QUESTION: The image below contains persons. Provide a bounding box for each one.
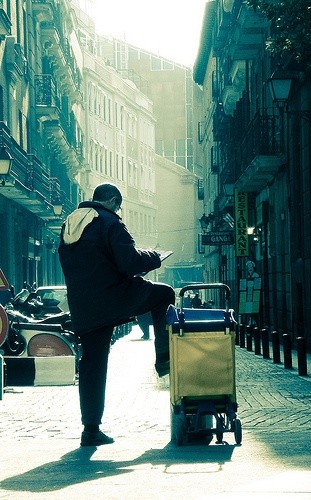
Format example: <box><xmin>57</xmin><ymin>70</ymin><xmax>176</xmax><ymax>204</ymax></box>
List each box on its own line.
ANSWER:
<box><xmin>192</xmin><ymin>293</ymin><xmax>202</xmax><ymax>309</ymax></box>
<box><xmin>58</xmin><ymin>184</ymin><xmax>176</xmax><ymax>448</ymax></box>
<box><xmin>137</xmin><ymin>312</ymin><xmax>150</xmax><ymax>339</ymax></box>
<box><xmin>183</xmin><ymin>295</ymin><xmax>192</xmax><ymax>308</ymax></box>
<box><xmin>245</xmin><ymin>260</ymin><xmax>259</xmax><ymax>278</ymax></box>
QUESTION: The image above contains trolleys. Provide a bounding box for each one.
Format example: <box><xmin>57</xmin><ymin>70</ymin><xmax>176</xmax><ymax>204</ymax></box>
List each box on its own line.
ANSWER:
<box><xmin>165</xmin><ymin>282</ymin><xmax>241</xmax><ymax>446</ymax></box>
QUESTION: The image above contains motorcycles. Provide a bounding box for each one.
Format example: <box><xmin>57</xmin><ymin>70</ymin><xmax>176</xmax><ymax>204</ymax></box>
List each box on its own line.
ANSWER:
<box><xmin>4</xmin><ymin>282</ymin><xmax>82</xmax><ymax>374</ymax></box>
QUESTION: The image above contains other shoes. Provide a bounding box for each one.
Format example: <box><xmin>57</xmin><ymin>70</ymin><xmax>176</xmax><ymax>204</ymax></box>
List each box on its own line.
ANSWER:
<box><xmin>80</xmin><ymin>430</ymin><xmax>113</xmax><ymax>446</ymax></box>
<box><xmin>156</xmin><ymin>361</ymin><xmax>169</xmax><ymax>377</ymax></box>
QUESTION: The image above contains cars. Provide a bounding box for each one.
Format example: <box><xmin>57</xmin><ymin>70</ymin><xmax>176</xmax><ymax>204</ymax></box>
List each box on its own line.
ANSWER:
<box><xmin>34</xmin><ymin>286</ymin><xmax>69</xmax><ymax>314</ymax></box>
